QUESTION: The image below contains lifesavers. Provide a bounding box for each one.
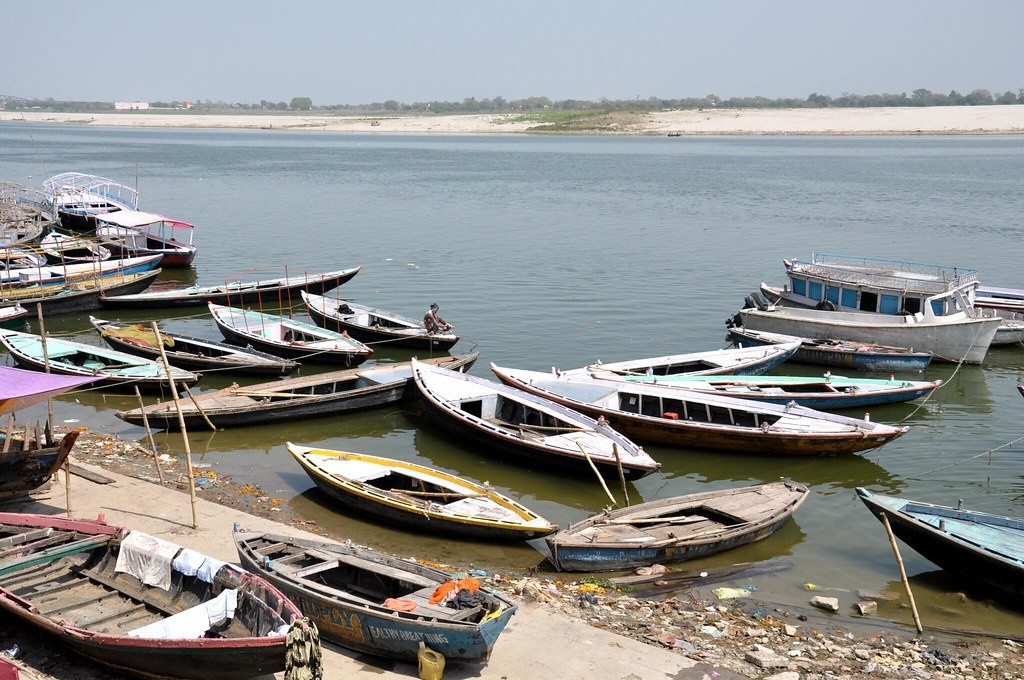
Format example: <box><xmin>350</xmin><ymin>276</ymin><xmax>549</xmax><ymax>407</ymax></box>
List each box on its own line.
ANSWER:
<box><xmin>896</xmin><ymin>311</ymin><xmax>913</xmax><ymax>316</ymax></box>
<box><xmin>815</xmin><ymin>300</ymin><xmax>836</xmax><ymax>311</ymax></box>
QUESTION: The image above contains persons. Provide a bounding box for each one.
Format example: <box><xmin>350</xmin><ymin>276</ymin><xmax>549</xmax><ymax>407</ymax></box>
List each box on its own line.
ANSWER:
<box><xmin>423</xmin><ymin>302</ymin><xmax>454</xmax><ymax>335</ymax></box>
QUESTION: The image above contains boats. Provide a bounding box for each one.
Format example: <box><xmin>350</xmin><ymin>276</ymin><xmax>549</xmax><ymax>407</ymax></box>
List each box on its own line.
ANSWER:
<box><xmin>411</xmin><ymin>356</ymin><xmax>663</xmax><ymax>482</ymax></box>
<box><xmin>0</xmin><ymin>512</ymin><xmax>311</xmax><ymax>680</ymax></box>
<box><xmin>0</xmin><ymin>171</ymin><xmax>164</xmax><ymax>330</ymax></box>
<box><xmin>98</xmin><ymin>263</ymin><xmax>362</xmax><ymax>310</ymax></box>
<box><xmin>88</xmin><ymin>315</ymin><xmax>302</xmax><ymax>377</ymax></box>
<box><xmin>301</xmin><ymin>289</ymin><xmax>461</xmax><ymax>352</ymax></box>
<box><xmin>855</xmin><ymin>486</ymin><xmax>1024</xmax><ymax>607</ymax></box>
<box><xmin>526</xmin><ymin>476</ymin><xmax>810</xmax><ymax>572</ymax></box>
<box><xmin>286</xmin><ymin>441</ymin><xmax>560</xmax><ymax>544</ymax></box>
<box><xmin>208</xmin><ymin>266</ymin><xmax>374</xmax><ymax>368</ymax></box>
<box><xmin>552</xmin><ymin>339</ymin><xmax>943</xmax><ymax>413</ymax></box>
<box><xmin>94</xmin><ymin>210</ymin><xmax>197</xmax><ymax>268</ymax></box>
<box><xmin>490</xmin><ymin>361</ymin><xmax>910</xmax><ymax>457</ymax></box>
<box><xmin>728</xmin><ymin>324</ymin><xmax>933</xmax><ymax>374</ymax></box>
<box><xmin>232</xmin><ymin>522</ymin><xmax>518</xmax><ymax>666</ymax></box>
<box><xmin>114</xmin><ymin>350</ymin><xmax>481</xmax><ymax>433</ymax></box>
<box><xmin>725</xmin><ymin>250</ymin><xmax>1024</xmax><ymax>366</ymax></box>
<box><xmin>0</xmin><ymin>366</ymin><xmax>110</xmax><ymax>500</ymax></box>
<box><xmin>0</xmin><ymin>329</ymin><xmax>203</xmax><ymax>396</ymax></box>
